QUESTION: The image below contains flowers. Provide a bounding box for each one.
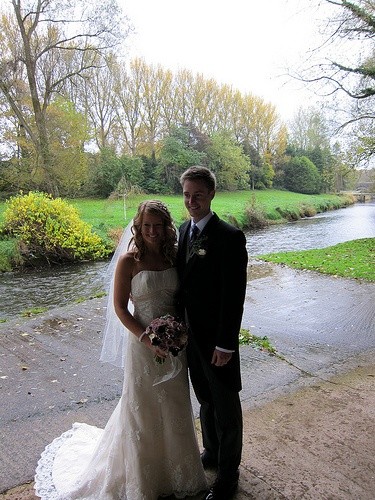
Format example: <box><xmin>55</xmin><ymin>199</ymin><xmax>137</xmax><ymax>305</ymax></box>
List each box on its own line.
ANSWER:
<box><xmin>196</xmin><ymin>248</ymin><xmax>207</xmax><ymax>257</ymax></box>
<box><xmin>139</xmin><ymin>314</ymin><xmax>188</xmax><ymax>363</ymax></box>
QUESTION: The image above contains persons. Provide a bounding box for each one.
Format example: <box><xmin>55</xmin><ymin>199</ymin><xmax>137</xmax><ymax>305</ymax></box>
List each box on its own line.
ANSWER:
<box><xmin>114</xmin><ymin>201</ymin><xmax>190</xmax><ymax>500</ymax></box>
<box><xmin>174</xmin><ymin>165</ymin><xmax>249</xmax><ymax>500</ymax></box>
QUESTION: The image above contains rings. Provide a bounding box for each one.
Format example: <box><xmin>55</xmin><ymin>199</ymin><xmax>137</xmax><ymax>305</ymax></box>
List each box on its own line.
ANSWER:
<box><xmin>222</xmin><ymin>362</ymin><xmax>227</xmax><ymax>364</ymax></box>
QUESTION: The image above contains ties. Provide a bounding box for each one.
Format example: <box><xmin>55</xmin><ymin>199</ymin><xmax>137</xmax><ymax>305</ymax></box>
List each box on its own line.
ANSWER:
<box><xmin>187</xmin><ymin>225</ymin><xmax>200</xmax><ymax>260</ymax></box>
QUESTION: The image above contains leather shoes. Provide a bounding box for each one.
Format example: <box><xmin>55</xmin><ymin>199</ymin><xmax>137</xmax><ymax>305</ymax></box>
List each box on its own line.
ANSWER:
<box><xmin>201</xmin><ymin>483</ymin><xmax>235</xmax><ymax>500</ymax></box>
<box><xmin>199</xmin><ymin>451</ymin><xmax>216</xmax><ymax>469</ymax></box>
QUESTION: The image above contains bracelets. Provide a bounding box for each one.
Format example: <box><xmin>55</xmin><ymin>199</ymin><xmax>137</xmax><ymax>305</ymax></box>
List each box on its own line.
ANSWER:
<box><xmin>139</xmin><ymin>331</ymin><xmax>147</xmax><ymax>343</ymax></box>
